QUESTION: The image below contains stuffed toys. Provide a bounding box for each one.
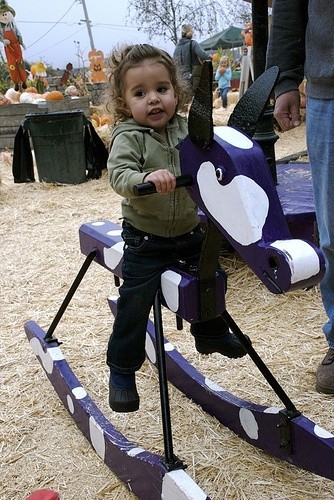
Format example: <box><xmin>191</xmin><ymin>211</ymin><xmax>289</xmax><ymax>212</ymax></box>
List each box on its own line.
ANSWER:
<box><xmin>0</xmin><ymin>0</ymin><xmax>29</xmax><ymax>92</ymax></box>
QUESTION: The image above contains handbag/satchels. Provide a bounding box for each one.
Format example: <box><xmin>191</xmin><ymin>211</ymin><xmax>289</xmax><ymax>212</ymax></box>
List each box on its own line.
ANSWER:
<box><xmin>181</xmin><ymin>72</ymin><xmax>193</xmax><ymax>87</ymax></box>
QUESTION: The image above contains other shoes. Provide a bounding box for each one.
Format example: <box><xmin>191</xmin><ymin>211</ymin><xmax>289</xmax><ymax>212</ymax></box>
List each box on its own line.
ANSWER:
<box><xmin>315</xmin><ymin>348</ymin><xmax>334</xmax><ymax>394</ymax></box>
<box><xmin>108</xmin><ymin>367</ymin><xmax>140</xmax><ymax>413</ymax></box>
<box><xmin>195</xmin><ymin>331</ymin><xmax>252</xmax><ymax>359</ymax></box>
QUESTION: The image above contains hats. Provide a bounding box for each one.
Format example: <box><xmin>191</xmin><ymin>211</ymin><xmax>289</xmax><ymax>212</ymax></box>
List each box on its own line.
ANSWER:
<box><xmin>181</xmin><ymin>24</ymin><xmax>195</xmax><ymax>38</ymax></box>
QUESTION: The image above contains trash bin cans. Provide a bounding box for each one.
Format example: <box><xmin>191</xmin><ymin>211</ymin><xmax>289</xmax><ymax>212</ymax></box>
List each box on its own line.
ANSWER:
<box><xmin>23</xmin><ymin>110</ymin><xmax>87</xmax><ymax>185</ymax></box>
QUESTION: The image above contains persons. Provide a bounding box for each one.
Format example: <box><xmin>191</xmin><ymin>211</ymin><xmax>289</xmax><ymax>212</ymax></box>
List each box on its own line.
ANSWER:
<box><xmin>103</xmin><ymin>42</ymin><xmax>252</xmax><ymax>413</ymax></box>
<box><xmin>263</xmin><ymin>0</ymin><xmax>334</xmax><ymax>396</ymax></box>
<box><xmin>174</xmin><ymin>23</ymin><xmax>214</xmax><ymax>87</ymax></box>
<box><xmin>215</xmin><ymin>56</ymin><xmax>232</xmax><ymax>108</ymax></box>
